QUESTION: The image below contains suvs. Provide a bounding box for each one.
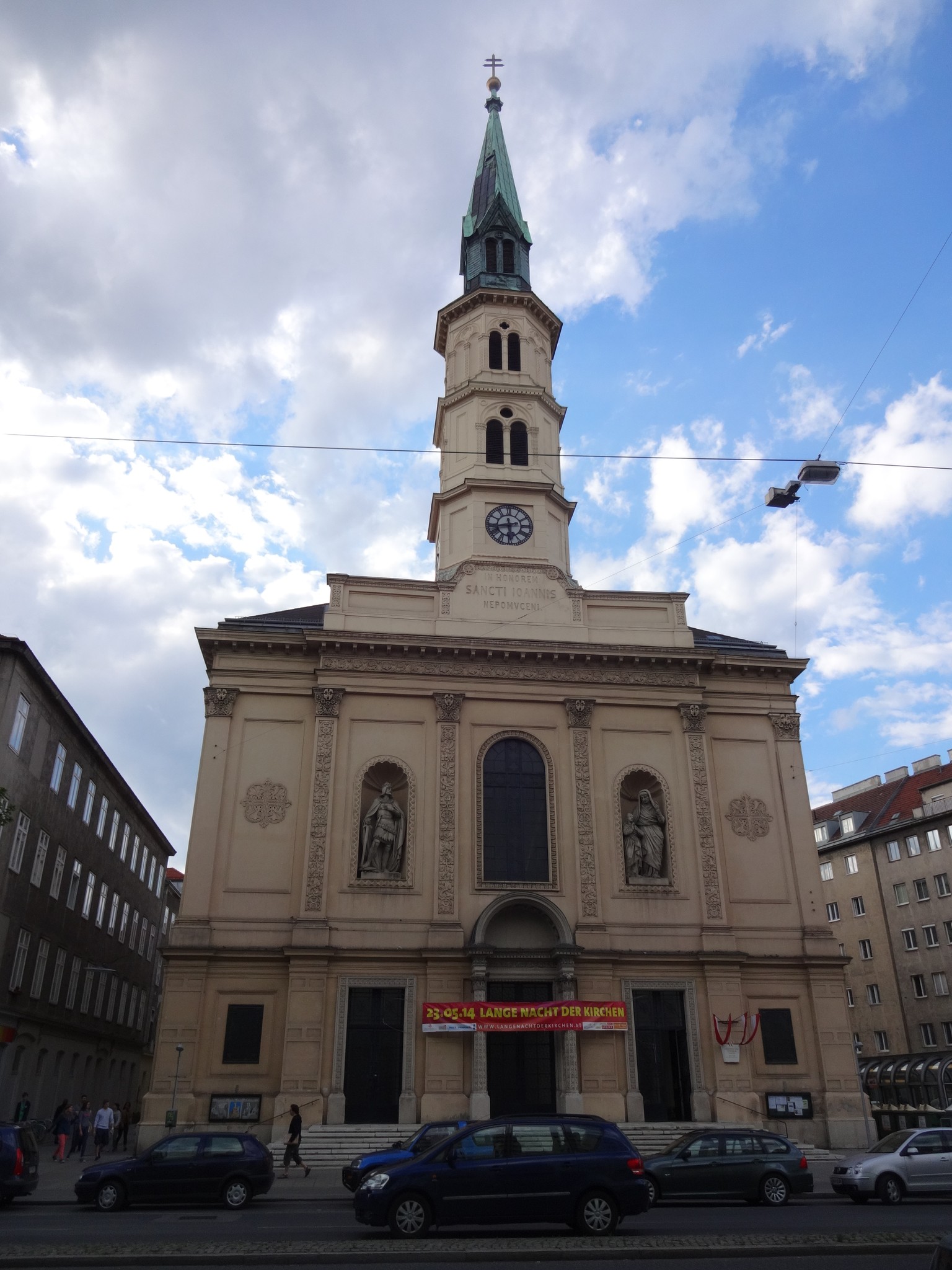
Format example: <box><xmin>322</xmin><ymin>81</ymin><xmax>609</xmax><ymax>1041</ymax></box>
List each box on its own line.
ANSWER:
<box><xmin>0</xmin><ymin>1122</ymin><xmax>40</xmax><ymax>1208</ymax></box>
<box><xmin>74</xmin><ymin>1131</ymin><xmax>275</xmax><ymax>1213</ymax></box>
<box><xmin>342</xmin><ymin>1120</ymin><xmax>496</xmax><ymax>1193</ymax></box>
<box><xmin>642</xmin><ymin>1128</ymin><xmax>813</xmax><ymax>1207</ymax></box>
<box><xmin>354</xmin><ymin>1114</ymin><xmax>650</xmax><ymax>1238</ymax></box>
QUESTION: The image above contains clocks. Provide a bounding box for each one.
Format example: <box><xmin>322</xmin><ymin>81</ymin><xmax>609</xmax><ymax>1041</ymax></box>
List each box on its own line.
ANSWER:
<box><xmin>485</xmin><ymin>505</ymin><xmax>533</xmax><ymax>546</ymax></box>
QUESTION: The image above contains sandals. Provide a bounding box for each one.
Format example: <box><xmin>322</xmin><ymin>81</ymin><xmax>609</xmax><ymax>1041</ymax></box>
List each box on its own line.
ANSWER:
<box><xmin>278</xmin><ymin>1173</ymin><xmax>288</xmax><ymax>1179</ymax></box>
<box><xmin>304</xmin><ymin>1166</ymin><xmax>311</xmax><ymax>1177</ymax></box>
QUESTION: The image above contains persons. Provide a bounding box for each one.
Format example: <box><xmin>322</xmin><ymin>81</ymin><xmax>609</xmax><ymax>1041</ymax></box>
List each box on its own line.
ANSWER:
<box><xmin>359</xmin><ymin>781</ymin><xmax>405</xmax><ymax>873</ymax></box>
<box><xmin>276</xmin><ymin>1104</ymin><xmax>311</xmax><ymax>1179</ymax></box>
<box><xmin>48</xmin><ymin>1094</ymin><xmax>132</xmax><ymax>1164</ymax></box>
<box><xmin>622</xmin><ymin>788</ymin><xmax>666</xmax><ymax>882</ymax></box>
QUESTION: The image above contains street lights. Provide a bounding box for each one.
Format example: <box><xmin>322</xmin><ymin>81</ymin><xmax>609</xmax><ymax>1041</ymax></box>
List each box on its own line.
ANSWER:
<box><xmin>168</xmin><ymin>1044</ymin><xmax>183</xmax><ymax>1135</ymax></box>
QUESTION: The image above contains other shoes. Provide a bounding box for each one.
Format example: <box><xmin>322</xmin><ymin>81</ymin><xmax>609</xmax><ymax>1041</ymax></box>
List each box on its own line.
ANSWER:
<box><xmin>124</xmin><ymin>1145</ymin><xmax>127</xmax><ymax>1151</ymax></box>
<box><xmin>99</xmin><ymin>1146</ymin><xmax>104</xmax><ymax>1152</ymax></box>
<box><xmin>80</xmin><ymin>1158</ymin><xmax>86</xmax><ymax>1162</ymax></box>
<box><xmin>52</xmin><ymin>1155</ymin><xmax>58</xmax><ymax>1162</ymax></box>
<box><xmin>77</xmin><ymin>1146</ymin><xmax>81</xmax><ymax>1151</ymax></box>
<box><xmin>59</xmin><ymin>1159</ymin><xmax>65</xmax><ymax>1163</ymax></box>
<box><xmin>94</xmin><ymin>1157</ymin><xmax>100</xmax><ymax>1163</ymax></box>
<box><xmin>66</xmin><ymin>1154</ymin><xmax>70</xmax><ymax>1160</ymax></box>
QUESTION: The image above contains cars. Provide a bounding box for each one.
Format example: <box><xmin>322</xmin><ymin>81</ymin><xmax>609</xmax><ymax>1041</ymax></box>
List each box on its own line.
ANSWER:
<box><xmin>829</xmin><ymin>1127</ymin><xmax>952</xmax><ymax>1207</ymax></box>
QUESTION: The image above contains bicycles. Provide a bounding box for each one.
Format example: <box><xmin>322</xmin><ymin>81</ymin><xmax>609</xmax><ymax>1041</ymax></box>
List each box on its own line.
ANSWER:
<box><xmin>29</xmin><ymin>1119</ymin><xmax>46</xmax><ymax>1143</ymax></box>
